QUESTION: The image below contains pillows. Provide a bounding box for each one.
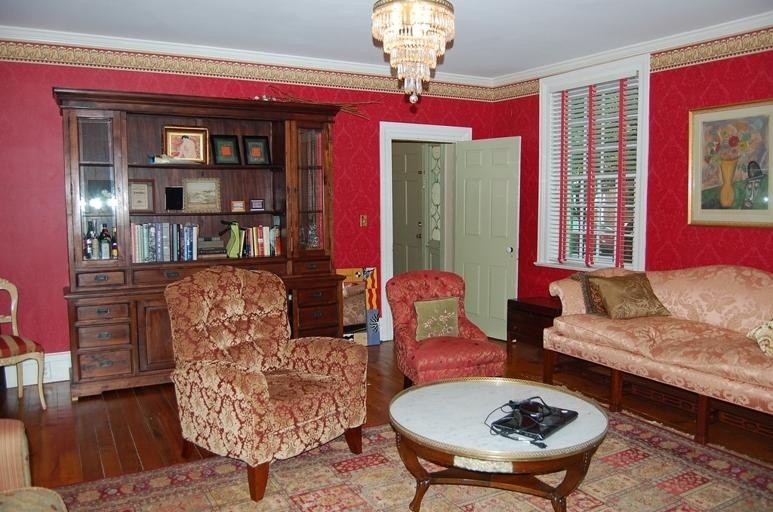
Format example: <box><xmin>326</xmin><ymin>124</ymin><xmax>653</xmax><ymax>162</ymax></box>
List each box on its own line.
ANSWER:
<box><xmin>590</xmin><ymin>272</ymin><xmax>670</xmax><ymax>318</ymax></box>
<box><xmin>413</xmin><ymin>296</ymin><xmax>461</xmax><ymax>341</ymax></box>
<box><xmin>581</xmin><ymin>272</ymin><xmax>604</xmax><ymax>314</ymax></box>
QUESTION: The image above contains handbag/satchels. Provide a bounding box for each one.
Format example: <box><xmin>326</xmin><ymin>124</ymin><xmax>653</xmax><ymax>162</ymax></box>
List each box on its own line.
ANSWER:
<box><xmin>218</xmin><ymin>220</ymin><xmax>239</xmax><ymax>259</ymax></box>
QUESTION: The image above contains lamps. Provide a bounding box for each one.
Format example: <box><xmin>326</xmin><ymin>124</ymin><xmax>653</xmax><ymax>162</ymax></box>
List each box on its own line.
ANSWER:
<box><xmin>371</xmin><ymin>0</ymin><xmax>454</xmax><ymax>105</ymax></box>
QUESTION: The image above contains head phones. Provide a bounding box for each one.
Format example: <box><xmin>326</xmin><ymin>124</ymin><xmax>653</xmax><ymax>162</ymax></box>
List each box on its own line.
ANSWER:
<box><xmin>511</xmin><ymin>395</ymin><xmax>552</xmax><ymax>430</ymax></box>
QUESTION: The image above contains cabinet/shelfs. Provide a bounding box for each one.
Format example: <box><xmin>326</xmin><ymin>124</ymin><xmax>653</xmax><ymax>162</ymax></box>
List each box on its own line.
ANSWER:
<box><xmin>45</xmin><ymin>86</ymin><xmax>345</xmax><ymax>401</ymax></box>
<box><xmin>507</xmin><ymin>297</ymin><xmax>562</xmax><ymax>387</ymax></box>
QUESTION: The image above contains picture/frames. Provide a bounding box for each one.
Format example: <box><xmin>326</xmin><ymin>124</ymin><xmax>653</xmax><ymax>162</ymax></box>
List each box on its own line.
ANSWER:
<box><xmin>687</xmin><ymin>99</ymin><xmax>773</xmax><ymax>228</ymax></box>
<box><xmin>242</xmin><ymin>135</ymin><xmax>272</xmax><ymax>164</ymax></box>
<box><xmin>181</xmin><ymin>178</ymin><xmax>221</xmax><ymax>212</ymax></box>
<box><xmin>249</xmin><ymin>198</ymin><xmax>265</xmax><ymax>211</ymax></box>
<box><xmin>211</xmin><ymin>134</ymin><xmax>241</xmax><ymax>164</ymax></box>
<box><xmin>231</xmin><ymin>200</ymin><xmax>245</xmax><ymax>212</ymax></box>
<box><xmin>166</xmin><ymin>186</ymin><xmax>183</xmax><ymax>210</ymax></box>
<box><xmin>129</xmin><ymin>179</ymin><xmax>156</xmax><ymax>214</ymax></box>
<box><xmin>161</xmin><ymin>124</ymin><xmax>209</xmax><ymax>164</ymax></box>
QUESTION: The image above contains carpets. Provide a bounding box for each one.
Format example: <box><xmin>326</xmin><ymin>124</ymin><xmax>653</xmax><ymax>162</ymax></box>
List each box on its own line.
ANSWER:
<box><xmin>44</xmin><ymin>379</ymin><xmax>773</xmax><ymax>512</ymax></box>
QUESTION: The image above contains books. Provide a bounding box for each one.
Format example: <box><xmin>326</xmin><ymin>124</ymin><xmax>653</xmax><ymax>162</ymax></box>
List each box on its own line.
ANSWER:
<box><xmin>130</xmin><ymin>218</ymin><xmax>197</xmax><ymax>261</ymax></box>
<box><xmin>226</xmin><ymin>221</ymin><xmax>282</xmax><ymax>256</ymax></box>
<box><xmin>197</xmin><ymin>235</ymin><xmax>227</xmax><ymax>258</ymax></box>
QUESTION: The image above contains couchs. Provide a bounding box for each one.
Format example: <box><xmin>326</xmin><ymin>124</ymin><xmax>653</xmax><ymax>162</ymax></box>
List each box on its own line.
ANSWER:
<box><xmin>386</xmin><ymin>271</ymin><xmax>507</xmax><ymax>388</ymax></box>
<box><xmin>1</xmin><ymin>420</ymin><xmax>68</xmax><ymax>512</ymax></box>
<box><xmin>164</xmin><ymin>264</ymin><xmax>368</xmax><ymax>502</ymax></box>
<box><xmin>543</xmin><ymin>265</ymin><xmax>773</xmax><ymax>461</ymax></box>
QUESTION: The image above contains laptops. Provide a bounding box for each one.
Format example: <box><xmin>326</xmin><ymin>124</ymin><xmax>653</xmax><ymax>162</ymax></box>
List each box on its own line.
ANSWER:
<box><xmin>491</xmin><ymin>401</ymin><xmax>578</xmax><ymax>440</ymax></box>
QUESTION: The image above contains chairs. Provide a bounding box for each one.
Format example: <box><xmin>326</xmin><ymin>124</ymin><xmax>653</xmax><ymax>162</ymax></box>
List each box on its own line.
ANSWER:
<box><xmin>0</xmin><ymin>279</ymin><xmax>47</xmax><ymax>411</ymax></box>
